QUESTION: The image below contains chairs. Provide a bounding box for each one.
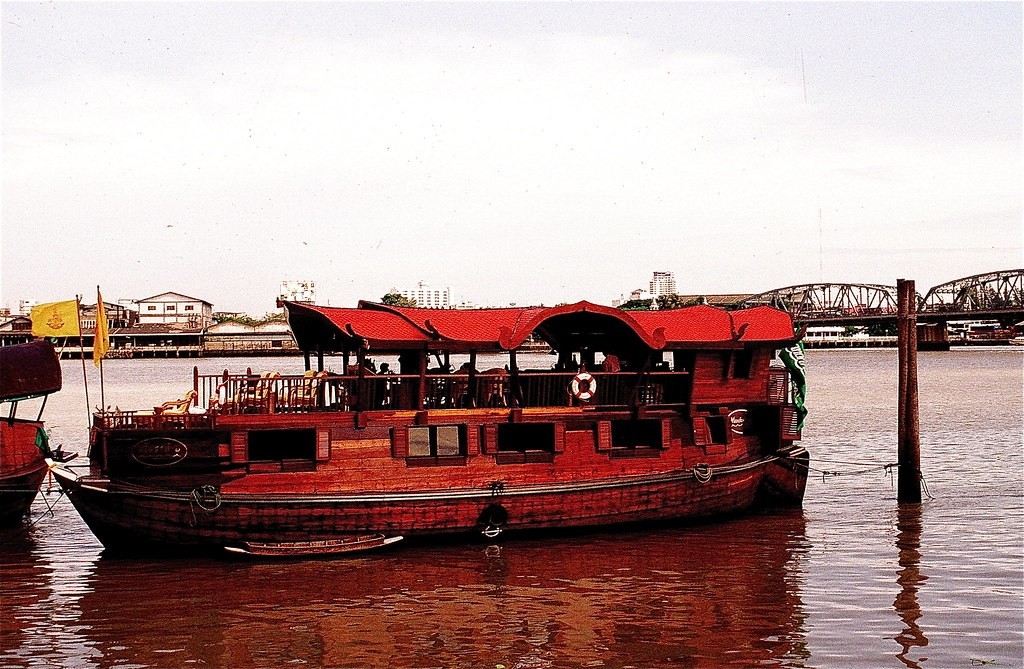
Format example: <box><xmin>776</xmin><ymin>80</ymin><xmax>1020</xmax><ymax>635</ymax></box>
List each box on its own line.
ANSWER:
<box><xmin>207</xmin><ymin>375</ymin><xmax>248</xmax><ymax>417</ymax></box>
<box><xmin>235</xmin><ymin>373</ymin><xmax>279</xmax><ymax>415</ymax></box>
<box><xmin>278</xmin><ymin>370</ymin><xmax>317</xmax><ymax>413</ymax></box>
<box><xmin>160</xmin><ymin>390</ymin><xmax>197</xmax><ymax>428</ymax></box>
<box><xmin>325</xmin><ymin>366</ymin><xmax>507</xmax><ymax>409</ymax></box>
<box><xmin>286</xmin><ymin>369</ymin><xmax>328</xmax><ymax>412</ymax></box>
<box><xmin>234</xmin><ymin>370</ymin><xmax>272</xmax><ymax>414</ymax></box>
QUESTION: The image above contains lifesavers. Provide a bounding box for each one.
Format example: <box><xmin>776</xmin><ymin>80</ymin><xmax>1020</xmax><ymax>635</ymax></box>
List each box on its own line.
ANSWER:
<box><xmin>571</xmin><ymin>373</ymin><xmax>597</xmax><ymax>401</ymax></box>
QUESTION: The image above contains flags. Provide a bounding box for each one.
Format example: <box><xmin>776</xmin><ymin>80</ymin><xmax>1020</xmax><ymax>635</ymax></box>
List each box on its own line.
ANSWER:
<box><xmin>29</xmin><ymin>300</ymin><xmax>81</xmax><ymax>337</ymax></box>
<box><xmin>93</xmin><ymin>292</ymin><xmax>109</xmax><ymax>369</ymax></box>
<box><xmin>779</xmin><ymin>321</ymin><xmax>808</xmax><ymax>431</ymax></box>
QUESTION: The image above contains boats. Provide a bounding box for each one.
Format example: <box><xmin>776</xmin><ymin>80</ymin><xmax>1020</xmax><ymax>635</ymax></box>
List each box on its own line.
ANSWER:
<box><xmin>44</xmin><ymin>298</ymin><xmax>811</xmax><ymax>549</ymax></box>
<box><xmin>1008</xmin><ymin>337</ymin><xmax>1024</xmax><ymax>346</ymax></box>
<box><xmin>223</xmin><ymin>532</ymin><xmax>404</xmax><ymax>563</ymax></box>
<box><xmin>0</xmin><ymin>340</ymin><xmax>62</xmax><ymax>532</ymax></box>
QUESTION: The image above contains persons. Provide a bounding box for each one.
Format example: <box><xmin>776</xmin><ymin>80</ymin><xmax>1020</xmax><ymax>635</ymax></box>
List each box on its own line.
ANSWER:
<box><xmin>240</xmin><ymin>367</ymin><xmax>257</xmax><ymax>394</ymax></box>
<box><xmin>601</xmin><ymin>349</ymin><xmax>621</xmax><ymax>378</ymax></box>
<box><xmin>365</xmin><ymin>359</ymin><xmax>508</xmax><ymax>411</ymax></box>
